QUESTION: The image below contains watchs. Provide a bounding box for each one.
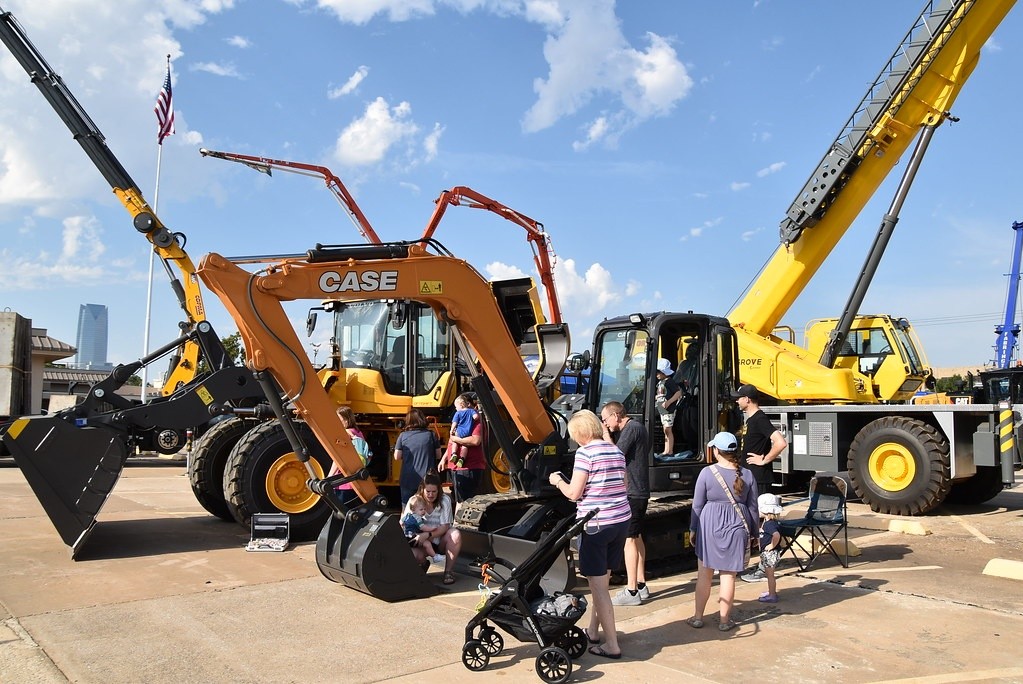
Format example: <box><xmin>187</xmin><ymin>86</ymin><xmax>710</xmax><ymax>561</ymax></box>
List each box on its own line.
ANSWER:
<box><xmin>555</xmin><ymin>478</ymin><xmax>563</xmax><ymax>489</ymax></box>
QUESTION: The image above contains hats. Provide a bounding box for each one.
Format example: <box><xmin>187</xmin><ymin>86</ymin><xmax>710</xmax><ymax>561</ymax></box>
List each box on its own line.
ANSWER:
<box><xmin>730</xmin><ymin>384</ymin><xmax>758</xmax><ymax>397</ymax></box>
<box><xmin>657</xmin><ymin>358</ymin><xmax>674</xmax><ymax>376</ymax></box>
<box><xmin>708</xmin><ymin>431</ymin><xmax>738</xmax><ymax>451</ymax></box>
<box><xmin>757</xmin><ymin>493</ymin><xmax>782</xmax><ymax>515</ymax></box>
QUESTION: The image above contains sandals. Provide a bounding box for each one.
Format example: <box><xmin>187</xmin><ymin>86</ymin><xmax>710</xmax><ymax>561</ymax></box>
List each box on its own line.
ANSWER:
<box><xmin>443</xmin><ymin>570</ymin><xmax>454</xmax><ymax>584</ymax></box>
<box><xmin>422</xmin><ymin>560</ymin><xmax>430</xmax><ymax>573</ymax></box>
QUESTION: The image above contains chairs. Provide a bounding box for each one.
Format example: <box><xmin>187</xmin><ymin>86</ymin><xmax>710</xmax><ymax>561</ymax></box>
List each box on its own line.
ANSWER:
<box><xmin>778</xmin><ymin>476</ymin><xmax>849</xmax><ymax>572</ymax></box>
<box><xmin>392</xmin><ymin>335</ymin><xmax>414</xmax><ymax>365</ymax></box>
<box><xmin>841</xmin><ymin>341</ymin><xmax>854</xmax><ymax>354</ymax></box>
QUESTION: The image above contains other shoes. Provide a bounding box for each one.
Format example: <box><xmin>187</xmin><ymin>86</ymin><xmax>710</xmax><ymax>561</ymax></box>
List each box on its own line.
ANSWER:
<box><xmin>686</xmin><ymin>617</ymin><xmax>704</xmax><ymax>628</ymax></box>
<box><xmin>449</xmin><ymin>452</ymin><xmax>457</xmax><ymax>463</ymax></box>
<box><xmin>758</xmin><ymin>592</ymin><xmax>778</xmax><ymax>603</ymax></box>
<box><xmin>719</xmin><ymin>619</ymin><xmax>735</xmax><ymax>630</ymax></box>
<box><xmin>456</xmin><ymin>457</ymin><xmax>464</xmax><ymax>468</ymax></box>
<box><xmin>433</xmin><ymin>554</ymin><xmax>445</xmax><ymax>563</ymax></box>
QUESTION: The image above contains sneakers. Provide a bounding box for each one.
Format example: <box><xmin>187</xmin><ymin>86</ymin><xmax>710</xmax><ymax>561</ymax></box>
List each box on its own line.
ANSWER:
<box><xmin>741</xmin><ymin>565</ymin><xmax>768</xmax><ymax>583</ymax></box>
<box><xmin>611</xmin><ymin>589</ymin><xmax>641</xmax><ymax>606</ymax></box>
<box><xmin>616</xmin><ymin>585</ymin><xmax>650</xmax><ymax>599</ymax></box>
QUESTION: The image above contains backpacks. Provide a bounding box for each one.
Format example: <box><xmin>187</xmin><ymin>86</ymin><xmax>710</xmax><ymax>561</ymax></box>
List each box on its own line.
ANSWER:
<box><xmin>345</xmin><ymin>428</ymin><xmax>372</xmax><ymax>468</ymax></box>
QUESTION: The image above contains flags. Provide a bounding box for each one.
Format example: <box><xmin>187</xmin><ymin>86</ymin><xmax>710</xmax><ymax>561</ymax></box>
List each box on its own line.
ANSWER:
<box><xmin>155</xmin><ymin>62</ymin><xmax>175</xmax><ymax>144</ymax></box>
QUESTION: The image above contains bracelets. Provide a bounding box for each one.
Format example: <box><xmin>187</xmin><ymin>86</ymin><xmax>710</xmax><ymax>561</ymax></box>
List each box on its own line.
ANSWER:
<box><xmin>428</xmin><ymin>532</ymin><xmax>432</xmax><ymax>538</ymax></box>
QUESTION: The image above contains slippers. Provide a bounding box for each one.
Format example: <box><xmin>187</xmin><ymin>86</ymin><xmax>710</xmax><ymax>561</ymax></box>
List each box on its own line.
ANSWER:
<box><xmin>581</xmin><ymin>626</ymin><xmax>601</xmax><ymax>643</ymax></box>
<box><xmin>588</xmin><ymin>644</ymin><xmax>621</xmax><ymax>659</ymax></box>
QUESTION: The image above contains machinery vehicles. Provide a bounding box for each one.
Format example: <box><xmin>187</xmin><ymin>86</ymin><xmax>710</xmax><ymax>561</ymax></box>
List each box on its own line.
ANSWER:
<box><xmin>0</xmin><ymin>7</ymin><xmax>548</xmax><ymax>560</ymax></box>
<box><xmin>195</xmin><ymin>239</ymin><xmax>744</xmax><ymax>604</ymax></box>
<box><xmin>623</xmin><ymin>0</ymin><xmax>1023</xmax><ymax>516</ymax></box>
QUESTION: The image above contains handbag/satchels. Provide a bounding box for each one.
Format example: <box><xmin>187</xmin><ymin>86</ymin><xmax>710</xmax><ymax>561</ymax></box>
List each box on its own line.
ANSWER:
<box><xmin>743</xmin><ymin>532</ymin><xmax>750</xmax><ymax>569</ymax></box>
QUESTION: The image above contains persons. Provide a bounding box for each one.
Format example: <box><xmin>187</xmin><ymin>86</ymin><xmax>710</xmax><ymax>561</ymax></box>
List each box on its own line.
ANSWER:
<box><xmin>399</xmin><ymin>470</ymin><xmax>462</xmax><ymax>585</ymax></box>
<box><xmin>689</xmin><ymin>431</ymin><xmax>759</xmax><ymax>630</ymax></box>
<box><xmin>437</xmin><ymin>391</ymin><xmax>487</xmax><ymax>504</ymax></box>
<box><xmin>730</xmin><ymin>384</ymin><xmax>788</xmax><ymax>582</ymax></box>
<box><xmin>395</xmin><ymin>408</ymin><xmax>439</xmax><ymax>516</ymax></box>
<box><xmin>327</xmin><ymin>406</ymin><xmax>371</xmax><ymax>508</ymax></box>
<box><xmin>655</xmin><ymin>343</ymin><xmax>700</xmax><ymax>457</ymax></box>
<box><xmin>549</xmin><ymin>409</ymin><xmax>633</xmax><ymax>658</ymax></box>
<box><xmin>756</xmin><ymin>493</ymin><xmax>783</xmax><ymax>602</ymax></box>
<box><xmin>600</xmin><ymin>401</ymin><xmax>649</xmax><ymax>606</ymax></box>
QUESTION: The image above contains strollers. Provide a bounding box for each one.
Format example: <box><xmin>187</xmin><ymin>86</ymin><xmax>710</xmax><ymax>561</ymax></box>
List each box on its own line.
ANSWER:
<box><xmin>459</xmin><ymin>507</ymin><xmax>603</xmax><ymax>684</ymax></box>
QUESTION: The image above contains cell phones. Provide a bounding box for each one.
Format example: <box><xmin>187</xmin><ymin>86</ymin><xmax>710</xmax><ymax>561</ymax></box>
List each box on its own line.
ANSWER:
<box><xmin>554</xmin><ymin>471</ymin><xmax>570</xmax><ymax>484</ymax></box>
